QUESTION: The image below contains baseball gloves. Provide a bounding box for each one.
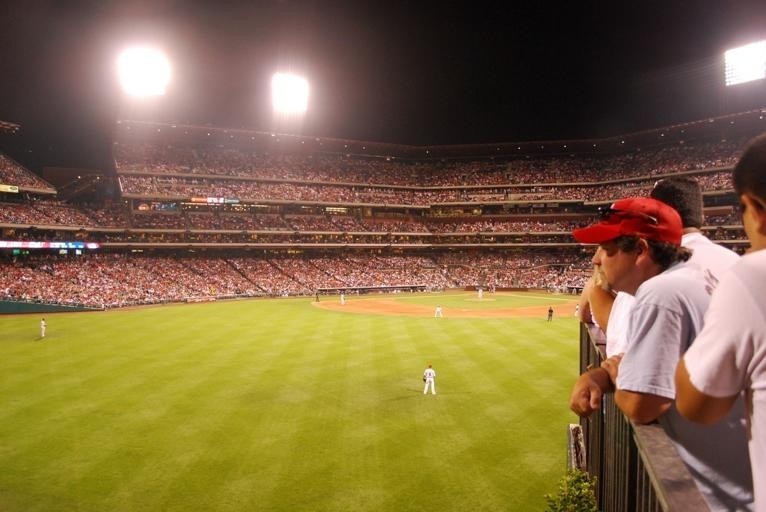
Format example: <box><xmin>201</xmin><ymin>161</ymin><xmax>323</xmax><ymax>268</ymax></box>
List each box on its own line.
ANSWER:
<box><xmin>423</xmin><ymin>379</ymin><xmax>426</xmax><ymax>382</ymax></box>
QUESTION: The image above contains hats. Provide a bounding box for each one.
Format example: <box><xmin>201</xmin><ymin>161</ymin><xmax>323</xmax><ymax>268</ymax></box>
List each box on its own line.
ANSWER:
<box><xmin>571</xmin><ymin>196</ymin><xmax>685</xmax><ymax>246</ymax></box>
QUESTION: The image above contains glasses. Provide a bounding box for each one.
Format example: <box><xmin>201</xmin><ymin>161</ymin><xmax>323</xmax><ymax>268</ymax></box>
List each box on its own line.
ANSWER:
<box><xmin>595</xmin><ymin>204</ymin><xmax>659</xmax><ymax>224</ymax></box>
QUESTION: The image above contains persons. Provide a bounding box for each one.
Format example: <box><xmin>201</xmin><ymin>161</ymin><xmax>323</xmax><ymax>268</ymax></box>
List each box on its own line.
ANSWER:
<box><xmin>567</xmin><ymin>196</ymin><xmax>756</xmax><ymax>511</ymax></box>
<box><xmin>422</xmin><ymin>364</ymin><xmax>436</xmax><ymax>394</ymax></box>
<box><xmin>1</xmin><ymin>105</ymin><xmax>765</xmax><ymax>341</ymax></box>
<box><xmin>674</xmin><ymin>133</ymin><xmax>765</xmax><ymax>512</ymax></box>
